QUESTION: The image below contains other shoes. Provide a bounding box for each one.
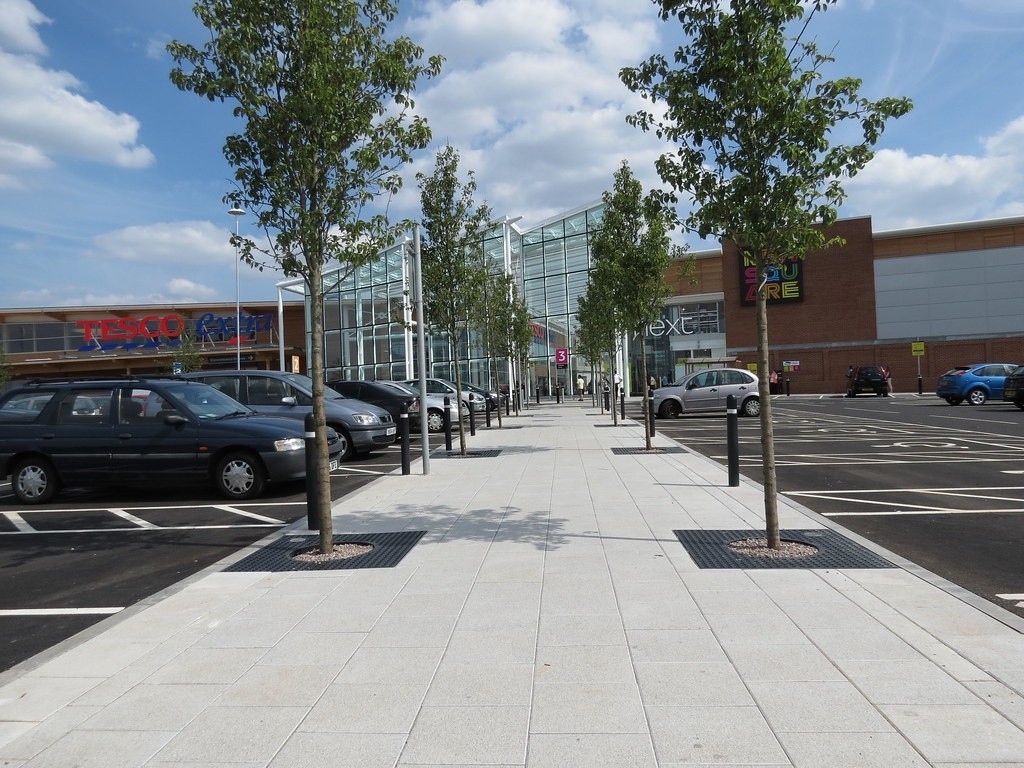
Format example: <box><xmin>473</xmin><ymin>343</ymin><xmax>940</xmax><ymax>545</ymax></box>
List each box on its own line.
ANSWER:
<box><xmin>578</xmin><ymin>399</ymin><xmax>583</xmax><ymax>401</ymax></box>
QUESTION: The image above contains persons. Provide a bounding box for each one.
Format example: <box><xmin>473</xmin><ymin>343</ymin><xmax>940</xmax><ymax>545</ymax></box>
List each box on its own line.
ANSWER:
<box><xmin>882</xmin><ymin>366</ymin><xmax>893</xmax><ymax>392</ymax></box>
<box><xmin>577</xmin><ymin>370</ymin><xmax>624</xmax><ymax>401</ymax></box>
<box><xmin>847</xmin><ymin>366</ymin><xmax>852</xmax><ymax>376</ymax></box>
<box><xmin>650</xmin><ymin>375</ymin><xmax>668</xmax><ymax>390</ymax></box>
<box><xmin>769</xmin><ymin>369</ymin><xmax>784</xmax><ymax>394</ymax></box>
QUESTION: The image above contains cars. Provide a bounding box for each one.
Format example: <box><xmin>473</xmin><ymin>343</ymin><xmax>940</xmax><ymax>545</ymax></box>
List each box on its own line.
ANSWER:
<box><xmin>1003</xmin><ymin>364</ymin><xmax>1024</xmax><ymax>408</ymax></box>
<box><xmin>936</xmin><ymin>364</ymin><xmax>1019</xmax><ymax>406</ymax></box>
<box><xmin>322</xmin><ymin>377</ymin><xmax>507</xmax><ymax>437</ymax></box>
<box><xmin>642</xmin><ymin>368</ymin><xmax>761</xmax><ymax>419</ymax></box>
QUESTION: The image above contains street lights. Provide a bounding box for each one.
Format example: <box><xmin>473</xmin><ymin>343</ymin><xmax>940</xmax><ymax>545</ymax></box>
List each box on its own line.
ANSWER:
<box><xmin>228</xmin><ymin>208</ymin><xmax>244</xmax><ymax>369</ymax></box>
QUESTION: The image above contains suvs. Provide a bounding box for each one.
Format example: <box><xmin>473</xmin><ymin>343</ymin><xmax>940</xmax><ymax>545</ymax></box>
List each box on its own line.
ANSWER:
<box><xmin>0</xmin><ymin>370</ymin><xmax>399</xmax><ymax>506</ymax></box>
<box><xmin>845</xmin><ymin>365</ymin><xmax>890</xmax><ymax>397</ymax></box>
